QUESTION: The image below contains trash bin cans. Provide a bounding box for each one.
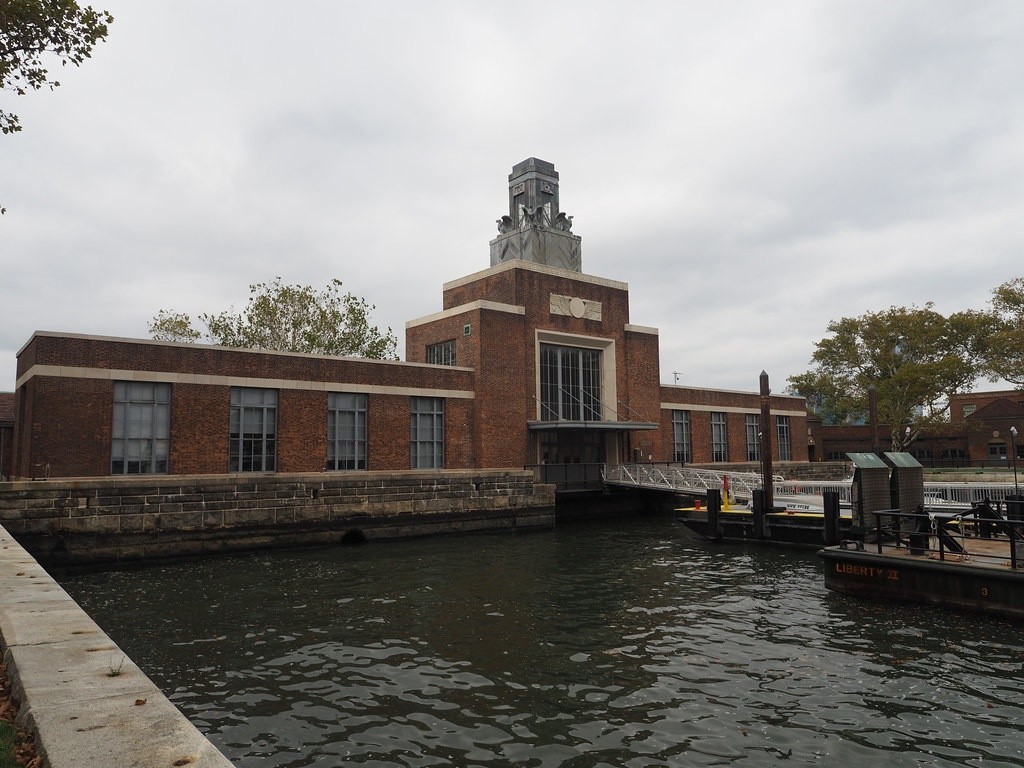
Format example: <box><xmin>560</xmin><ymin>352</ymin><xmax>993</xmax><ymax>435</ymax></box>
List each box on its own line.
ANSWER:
<box><xmin>845</xmin><ymin>452</ymin><xmax>895</xmax><ymax>542</ymax></box>
<box><xmin>882</xmin><ymin>452</ymin><xmax>925</xmax><ymax>538</ymax></box>
